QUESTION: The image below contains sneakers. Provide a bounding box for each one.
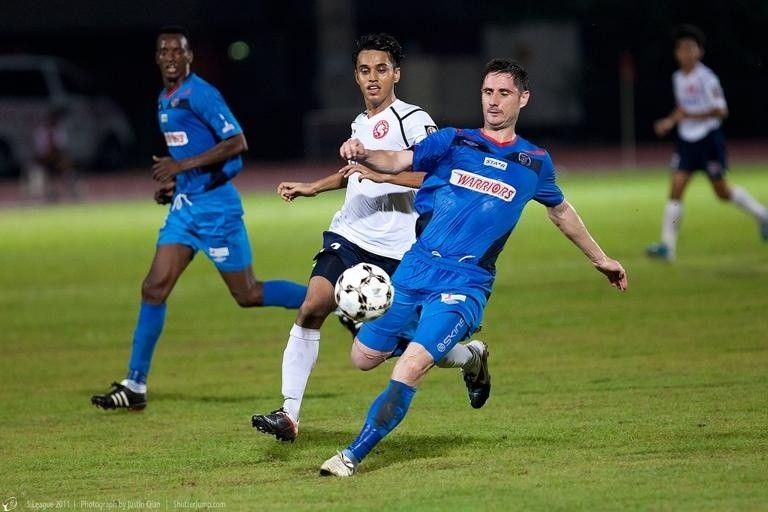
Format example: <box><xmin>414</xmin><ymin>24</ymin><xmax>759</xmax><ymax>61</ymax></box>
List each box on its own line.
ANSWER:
<box><xmin>251</xmin><ymin>407</ymin><xmax>299</xmax><ymax>443</ymax></box>
<box><xmin>91</xmin><ymin>381</ymin><xmax>147</xmax><ymax>411</ymax></box>
<box><xmin>642</xmin><ymin>242</ymin><xmax>676</xmax><ymax>258</ymax></box>
<box><xmin>460</xmin><ymin>340</ymin><xmax>490</xmax><ymax>408</ymax></box>
<box><xmin>319</xmin><ymin>449</ymin><xmax>359</xmax><ymax>478</ymax></box>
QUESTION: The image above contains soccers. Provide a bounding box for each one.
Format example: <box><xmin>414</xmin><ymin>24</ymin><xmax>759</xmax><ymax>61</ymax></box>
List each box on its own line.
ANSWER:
<box><xmin>334</xmin><ymin>263</ymin><xmax>394</xmax><ymax>322</ymax></box>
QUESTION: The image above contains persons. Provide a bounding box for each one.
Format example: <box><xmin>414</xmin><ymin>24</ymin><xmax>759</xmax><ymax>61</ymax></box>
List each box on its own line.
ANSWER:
<box><xmin>643</xmin><ymin>27</ymin><xmax>768</xmax><ymax>263</ymax></box>
<box><xmin>319</xmin><ymin>56</ymin><xmax>627</xmax><ymax>478</ymax></box>
<box><xmin>251</xmin><ymin>32</ymin><xmax>439</xmax><ymax>444</ymax></box>
<box><xmin>90</xmin><ymin>25</ymin><xmax>367</xmax><ymax>411</ymax></box>
